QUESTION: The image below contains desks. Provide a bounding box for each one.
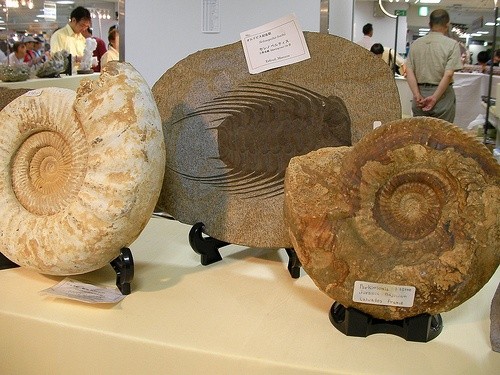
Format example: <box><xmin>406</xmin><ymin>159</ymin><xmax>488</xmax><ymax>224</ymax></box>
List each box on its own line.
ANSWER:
<box><xmin>0</xmin><ymin>214</ymin><xmax>500</xmax><ymax>375</ymax></box>
<box><xmin>0</xmin><ymin>72</ymin><xmax>101</xmax><ymax>90</ymax></box>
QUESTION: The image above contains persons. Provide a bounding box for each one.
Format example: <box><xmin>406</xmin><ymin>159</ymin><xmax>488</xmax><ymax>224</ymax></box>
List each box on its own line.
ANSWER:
<box><xmin>50</xmin><ymin>6</ymin><xmax>98</xmax><ymax>70</ymax></box>
<box><xmin>371</xmin><ymin>42</ymin><xmax>406</xmax><ymax>77</ymax></box>
<box><xmin>406</xmin><ymin>9</ymin><xmax>461</xmax><ymax>123</ymax></box>
<box><xmin>81</xmin><ymin>23</ymin><xmax>120</xmax><ymax>72</ymax></box>
<box><xmin>357</xmin><ymin>23</ymin><xmax>375</xmax><ymax>50</ymax></box>
<box><xmin>0</xmin><ymin>31</ymin><xmax>51</xmax><ymax>63</ymax></box>
<box><xmin>468</xmin><ymin>49</ymin><xmax>500</xmax><ymax>75</ymax></box>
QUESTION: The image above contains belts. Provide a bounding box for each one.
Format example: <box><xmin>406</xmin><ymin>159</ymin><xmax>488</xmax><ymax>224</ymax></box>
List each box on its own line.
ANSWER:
<box><xmin>417</xmin><ymin>83</ymin><xmax>438</xmax><ymax>86</ymax></box>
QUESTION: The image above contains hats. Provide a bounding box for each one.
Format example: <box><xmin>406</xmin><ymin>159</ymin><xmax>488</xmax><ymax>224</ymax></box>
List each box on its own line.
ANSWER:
<box><xmin>23</xmin><ymin>36</ymin><xmax>37</xmax><ymax>42</ymax></box>
<box><xmin>33</xmin><ymin>36</ymin><xmax>44</xmax><ymax>42</ymax></box>
